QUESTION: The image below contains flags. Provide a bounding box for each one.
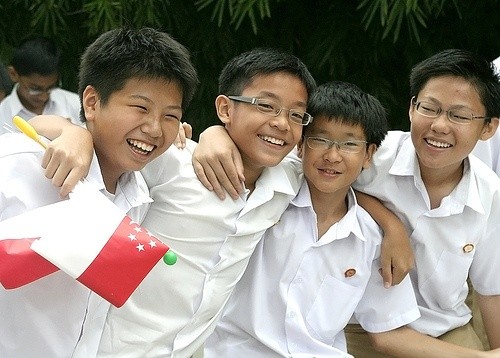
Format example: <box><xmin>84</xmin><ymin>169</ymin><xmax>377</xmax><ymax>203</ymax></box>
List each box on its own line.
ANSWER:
<box><xmin>0</xmin><ymin>180</ymin><xmax>87</xmax><ymax>291</ymax></box>
<box><xmin>30</xmin><ymin>182</ymin><xmax>170</xmax><ymax>310</ymax></box>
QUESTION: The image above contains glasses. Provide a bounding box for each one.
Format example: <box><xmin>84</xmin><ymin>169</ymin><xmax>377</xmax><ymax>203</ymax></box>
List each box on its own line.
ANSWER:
<box><xmin>228</xmin><ymin>96</ymin><xmax>314</xmax><ymax>125</ymax></box>
<box><xmin>412</xmin><ymin>96</ymin><xmax>489</xmax><ymax>124</ymax></box>
<box><xmin>19</xmin><ymin>76</ymin><xmax>62</xmax><ymax>96</ymax></box>
<box><xmin>302</xmin><ymin>136</ymin><xmax>368</xmax><ymax>153</ymax></box>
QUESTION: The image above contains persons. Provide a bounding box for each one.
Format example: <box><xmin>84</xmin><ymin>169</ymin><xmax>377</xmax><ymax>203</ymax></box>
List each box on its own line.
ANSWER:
<box><xmin>171</xmin><ymin>79</ymin><xmax>500</xmax><ymax>358</ymax></box>
<box><xmin>190</xmin><ymin>48</ymin><xmax>500</xmax><ymax>358</ymax></box>
<box><xmin>0</xmin><ymin>25</ymin><xmax>204</xmax><ymax>358</ymax></box>
<box><xmin>469</xmin><ymin>54</ymin><xmax>500</xmax><ymax>177</ymax></box>
<box><xmin>0</xmin><ymin>33</ymin><xmax>89</xmax><ymax>140</ymax></box>
<box><xmin>27</xmin><ymin>46</ymin><xmax>413</xmax><ymax>358</ymax></box>
